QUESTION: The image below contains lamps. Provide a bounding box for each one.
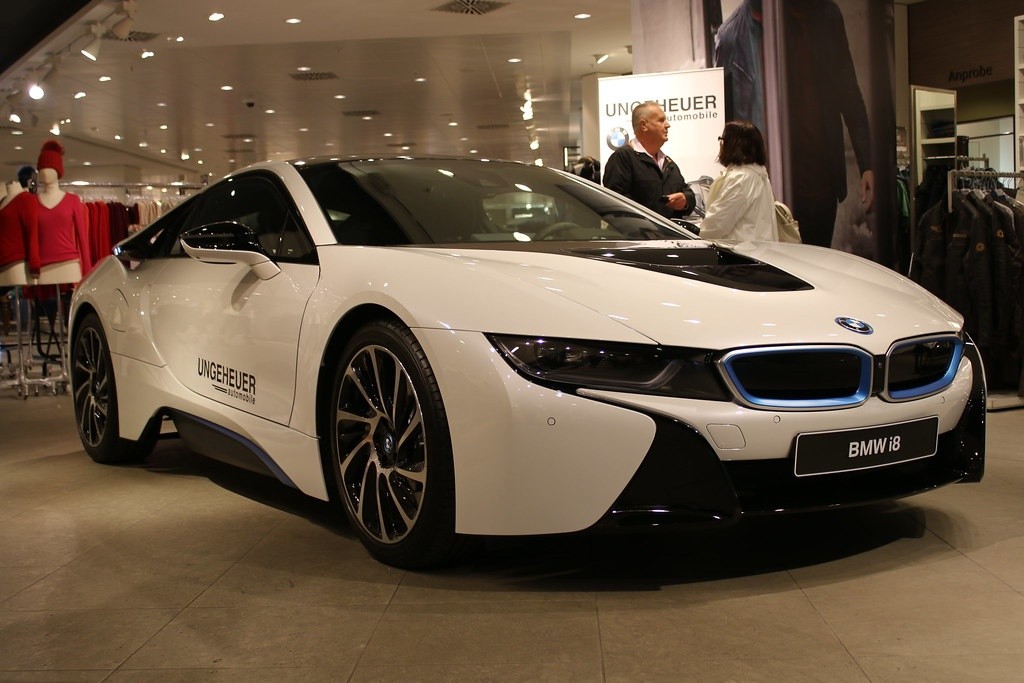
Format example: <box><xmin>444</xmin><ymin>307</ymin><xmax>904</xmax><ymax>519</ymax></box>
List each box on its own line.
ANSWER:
<box><xmin>27</xmin><ymin>0</ymin><xmax>138</xmax><ymax>101</ymax></box>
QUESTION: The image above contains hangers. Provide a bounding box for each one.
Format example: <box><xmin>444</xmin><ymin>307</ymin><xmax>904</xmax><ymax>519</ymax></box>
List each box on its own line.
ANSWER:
<box><xmin>951</xmin><ymin>169</ymin><xmax>1024</xmax><ymax>202</ymax></box>
<box><xmin>55</xmin><ymin>180</ymin><xmax>208</xmax><ymax>204</ymax></box>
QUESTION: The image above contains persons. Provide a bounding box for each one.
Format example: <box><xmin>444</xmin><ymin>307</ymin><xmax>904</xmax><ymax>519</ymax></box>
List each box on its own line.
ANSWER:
<box><xmin>714</xmin><ymin>0</ymin><xmax>875</xmax><ymax>248</ymax></box>
<box><xmin>698</xmin><ymin>122</ymin><xmax>778</xmax><ymax>243</ymax></box>
<box><xmin>603</xmin><ymin>101</ymin><xmax>696</xmax><ymax>219</ymax></box>
<box><xmin>0</xmin><ymin>140</ymin><xmax>92</xmax><ymax>285</ymax></box>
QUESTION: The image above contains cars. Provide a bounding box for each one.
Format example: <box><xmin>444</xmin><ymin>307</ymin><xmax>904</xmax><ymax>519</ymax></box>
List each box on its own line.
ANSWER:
<box><xmin>64</xmin><ymin>152</ymin><xmax>989</xmax><ymax>573</ymax></box>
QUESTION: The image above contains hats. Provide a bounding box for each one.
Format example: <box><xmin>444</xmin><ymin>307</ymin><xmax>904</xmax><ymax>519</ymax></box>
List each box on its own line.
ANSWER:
<box><xmin>37</xmin><ymin>141</ymin><xmax>64</xmax><ymax>179</ymax></box>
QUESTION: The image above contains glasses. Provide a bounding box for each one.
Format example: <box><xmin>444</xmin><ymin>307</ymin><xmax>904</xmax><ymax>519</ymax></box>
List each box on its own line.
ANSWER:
<box><xmin>718</xmin><ymin>137</ymin><xmax>725</xmax><ymax>142</ymax></box>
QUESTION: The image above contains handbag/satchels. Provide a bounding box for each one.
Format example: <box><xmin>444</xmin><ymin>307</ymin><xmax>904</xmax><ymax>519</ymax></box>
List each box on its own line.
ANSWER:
<box><xmin>774</xmin><ymin>201</ymin><xmax>802</xmax><ymax>244</ymax></box>
